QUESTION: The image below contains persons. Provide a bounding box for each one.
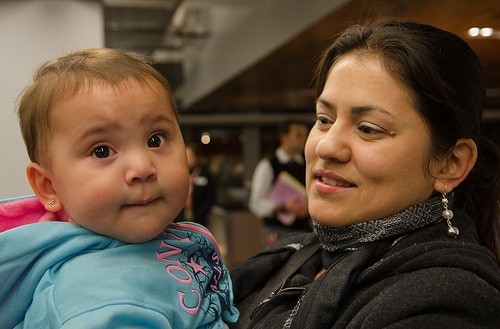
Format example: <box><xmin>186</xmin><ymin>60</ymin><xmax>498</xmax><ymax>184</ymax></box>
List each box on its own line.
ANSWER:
<box><xmin>0</xmin><ymin>19</ymin><xmax>500</xmax><ymax>329</ymax></box>
